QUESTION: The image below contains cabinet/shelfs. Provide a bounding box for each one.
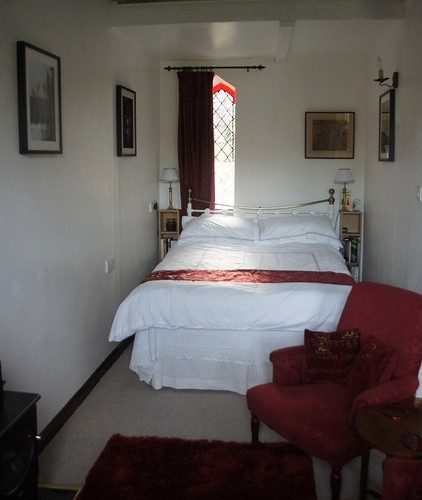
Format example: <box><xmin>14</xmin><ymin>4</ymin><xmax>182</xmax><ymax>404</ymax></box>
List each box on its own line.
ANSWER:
<box><xmin>0</xmin><ymin>389</ymin><xmax>42</xmax><ymax>500</ymax></box>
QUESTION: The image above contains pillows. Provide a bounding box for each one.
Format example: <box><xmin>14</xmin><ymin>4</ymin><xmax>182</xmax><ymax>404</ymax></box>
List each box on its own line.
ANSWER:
<box><xmin>177</xmin><ymin>213</ymin><xmax>260</xmax><ymax>243</ymax></box>
<box><xmin>346</xmin><ymin>335</ymin><xmax>398</xmax><ymax>396</ymax></box>
<box><xmin>303</xmin><ymin>327</ymin><xmax>362</xmax><ymax>384</ymax></box>
<box><xmin>258</xmin><ymin>213</ymin><xmax>344</xmax><ymax>249</ymax></box>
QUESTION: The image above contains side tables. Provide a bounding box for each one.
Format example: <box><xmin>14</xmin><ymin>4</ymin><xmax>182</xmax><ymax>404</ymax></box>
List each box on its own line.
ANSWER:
<box><xmin>355</xmin><ymin>397</ymin><xmax>422</xmax><ymax>500</ymax></box>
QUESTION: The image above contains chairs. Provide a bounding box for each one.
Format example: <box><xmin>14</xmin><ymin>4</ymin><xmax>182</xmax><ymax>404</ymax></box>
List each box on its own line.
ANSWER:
<box><xmin>246</xmin><ymin>280</ymin><xmax>422</xmax><ymax>500</ymax></box>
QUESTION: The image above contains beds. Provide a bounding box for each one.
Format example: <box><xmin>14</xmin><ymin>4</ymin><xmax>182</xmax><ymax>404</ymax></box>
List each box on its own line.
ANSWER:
<box><xmin>108</xmin><ymin>186</ymin><xmax>358</xmax><ymax>396</ymax></box>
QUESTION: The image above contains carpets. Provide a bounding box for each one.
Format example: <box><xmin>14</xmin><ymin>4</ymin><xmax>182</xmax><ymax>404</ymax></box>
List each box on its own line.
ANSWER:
<box><xmin>71</xmin><ymin>434</ymin><xmax>316</xmax><ymax>500</ymax></box>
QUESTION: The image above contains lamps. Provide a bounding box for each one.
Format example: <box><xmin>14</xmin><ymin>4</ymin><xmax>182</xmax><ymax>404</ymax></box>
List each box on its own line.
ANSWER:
<box><xmin>372</xmin><ymin>68</ymin><xmax>399</xmax><ymax>88</ymax></box>
<box><xmin>159</xmin><ymin>168</ymin><xmax>181</xmax><ymax>211</ymax></box>
<box><xmin>334</xmin><ymin>167</ymin><xmax>355</xmax><ymax>213</ymax></box>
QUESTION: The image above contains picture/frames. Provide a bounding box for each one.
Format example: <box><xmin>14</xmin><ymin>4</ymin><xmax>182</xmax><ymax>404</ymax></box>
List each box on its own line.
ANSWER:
<box><xmin>115</xmin><ymin>84</ymin><xmax>137</xmax><ymax>158</ymax></box>
<box><xmin>16</xmin><ymin>40</ymin><xmax>64</xmax><ymax>156</ymax></box>
<box><xmin>304</xmin><ymin>111</ymin><xmax>355</xmax><ymax>159</ymax></box>
<box><xmin>378</xmin><ymin>88</ymin><xmax>395</xmax><ymax>163</ymax></box>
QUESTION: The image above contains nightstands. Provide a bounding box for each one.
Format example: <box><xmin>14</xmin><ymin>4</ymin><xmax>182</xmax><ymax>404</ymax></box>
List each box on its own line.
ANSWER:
<box><xmin>339</xmin><ymin>210</ymin><xmax>363</xmax><ymax>284</ymax></box>
<box><xmin>157</xmin><ymin>208</ymin><xmax>181</xmax><ymax>263</ymax></box>
